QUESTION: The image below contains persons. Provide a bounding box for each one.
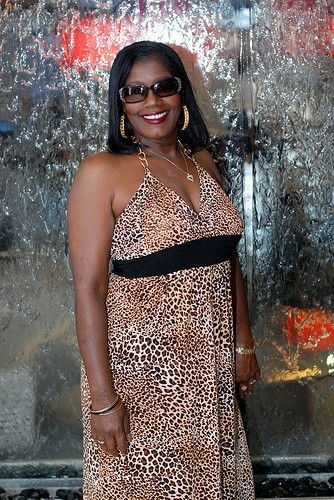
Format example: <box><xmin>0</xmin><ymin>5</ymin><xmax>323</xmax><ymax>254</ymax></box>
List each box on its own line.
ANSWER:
<box><xmin>66</xmin><ymin>38</ymin><xmax>264</xmax><ymax>500</ymax></box>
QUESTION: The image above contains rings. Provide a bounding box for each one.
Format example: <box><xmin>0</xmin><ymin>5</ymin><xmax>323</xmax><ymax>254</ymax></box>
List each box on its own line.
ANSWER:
<box><xmin>249</xmin><ymin>380</ymin><xmax>256</xmax><ymax>385</ymax></box>
<box><xmin>97</xmin><ymin>440</ymin><xmax>105</xmax><ymax>444</ymax></box>
<box><xmin>241</xmin><ymin>385</ymin><xmax>248</xmax><ymax>390</ymax></box>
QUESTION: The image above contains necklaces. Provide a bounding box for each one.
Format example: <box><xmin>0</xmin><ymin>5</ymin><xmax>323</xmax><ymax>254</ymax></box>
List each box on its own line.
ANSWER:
<box><xmin>138</xmin><ymin>141</ymin><xmax>195</xmax><ymax>182</ymax></box>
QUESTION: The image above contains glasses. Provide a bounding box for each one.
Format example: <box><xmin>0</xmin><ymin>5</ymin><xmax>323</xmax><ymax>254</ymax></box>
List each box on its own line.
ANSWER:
<box><xmin>118</xmin><ymin>77</ymin><xmax>183</xmax><ymax>104</ymax></box>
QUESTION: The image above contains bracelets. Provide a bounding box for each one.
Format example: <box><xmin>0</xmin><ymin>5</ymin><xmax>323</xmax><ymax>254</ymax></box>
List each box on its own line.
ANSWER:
<box><xmin>89</xmin><ymin>396</ymin><xmax>123</xmax><ymax>416</ymax></box>
<box><xmin>234</xmin><ymin>346</ymin><xmax>257</xmax><ymax>355</ymax></box>
